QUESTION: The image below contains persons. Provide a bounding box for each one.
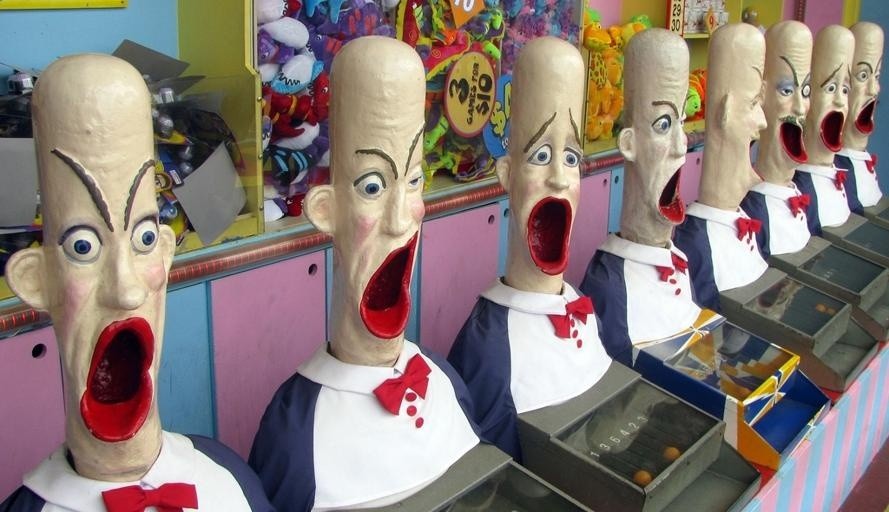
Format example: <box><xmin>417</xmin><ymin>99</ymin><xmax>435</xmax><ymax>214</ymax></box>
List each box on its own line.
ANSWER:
<box><xmin>0</xmin><ymin>0</ymin><xmax>889</xmax><ymax>512</ymax></box>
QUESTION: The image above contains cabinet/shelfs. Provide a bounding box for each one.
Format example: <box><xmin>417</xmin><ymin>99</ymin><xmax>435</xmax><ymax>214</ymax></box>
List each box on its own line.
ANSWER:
<box><xmin>678</xmin><ymin>146</ymin><xmax>704</xmax><ymax>207</ymax></box>
<box><xmin>419</xmin><ymin>199</ymin><xmax>510</xmax><ymax>360</ymax></box>
<box><xmin>207</xmin><ymin>226</ymin><xmax>420</xmax><ymax>464</ymax></box>
<box><xmin>563</xmin><ymin>164</ymin><xmax>624</xmax><ymax>288</ymax></box>
<box><xmin>0</xmin><ymin>283</ymin><xmax>215</xmax><ymax>512</ymax></box>
